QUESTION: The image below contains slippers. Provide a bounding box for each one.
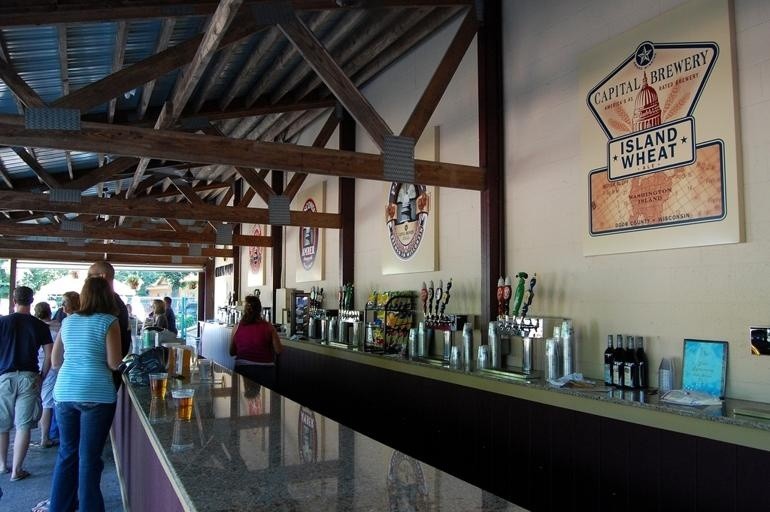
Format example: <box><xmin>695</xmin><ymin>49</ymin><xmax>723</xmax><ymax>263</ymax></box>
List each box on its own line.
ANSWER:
<box><xmin>9</xmin><ymin>470</ymin><xmax>30</xmax><ymax>483</ymax></box>
<box><xmin>40</xmin><ymin>439</ymin><xmax>59</xmax><ymax>449</ymax></box>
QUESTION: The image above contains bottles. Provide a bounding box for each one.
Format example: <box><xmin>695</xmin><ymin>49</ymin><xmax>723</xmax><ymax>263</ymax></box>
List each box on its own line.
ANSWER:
<box><xmin>144</xmin><ymin>330</ymin><xmax>151</xmax><ymax>348</ymax></box>
<box><xmin>658</xmin><ymin>359</ymin><xmax>673</xmax><ymax>396</ymax></box>
<box><xmin>604</xmin><ymin>334</ymin><xmax>649</xmax><ymax>391</ymax></box>
<box><xmin>307</xmin><ymin>316</ymin><xmax>338</xmax><ymax>341</ymax></box>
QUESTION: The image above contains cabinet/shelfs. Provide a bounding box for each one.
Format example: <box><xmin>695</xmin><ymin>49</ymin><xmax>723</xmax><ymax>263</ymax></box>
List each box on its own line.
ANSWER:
<box><xmin>362</xmin><ymin>292</ymin><xmax>416</xmax><ymax>356</ymax></box>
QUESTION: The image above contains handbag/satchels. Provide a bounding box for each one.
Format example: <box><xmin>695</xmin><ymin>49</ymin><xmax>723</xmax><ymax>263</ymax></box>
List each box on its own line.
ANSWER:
<box><xmin>117</xmin><ymin>346</ymin><xmax>169</xmax><ymax>385</ymax></box>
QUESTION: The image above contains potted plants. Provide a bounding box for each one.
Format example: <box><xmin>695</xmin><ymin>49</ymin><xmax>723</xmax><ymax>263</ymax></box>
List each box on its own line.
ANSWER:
<box><xmin>124</xmin><ymin>272</ymin><xmax>145</xmax><ymax>289</ymax></box>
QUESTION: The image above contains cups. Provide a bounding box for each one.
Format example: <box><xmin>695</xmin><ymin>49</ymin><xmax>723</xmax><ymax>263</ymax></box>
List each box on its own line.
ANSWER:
<box><xmin>450</xmin><ymin>321</ymin><xmax>500</xmax><ymax>368</ymax></box>
<box><xmin>197</xmin><ymin>358</ymin><xmax>210</xmax><ymax>379</ymax></box>
<box><xmin>172</xmin><ymin>388</ymin><xmax>195</xmax><ymax>423</ymax></box>
<box><xmin>149</xmin><ymin>401</ymin><xmax>170</xmax><ymax>423</ymax></box>
<box><xmin>171</xmin><ymin>420</ymin><xmax>196</xmax><ymax>451</ymax></box>
<box><xmin>149</xmin><ymin>373</ymin><xmax>168</xmax><ymax>401</ymax></box>
<box><xmin>132</xmin><ymin>335</ymin><xmax>143</xmax><ymax>351</ymax></box>
<box><xmin>162</xmin><ymin>342</ymin><xmax>196</xmax><ymax>375</ymax></box>
<box><xmin>544</xmin><ymin>320</ymin><xmax>574</xmax><ymax>381</ymax></box>
<box><xmin>408</xmin><ymin>321</ymin><xmax>434</xmax><ymax>360</ymax></box>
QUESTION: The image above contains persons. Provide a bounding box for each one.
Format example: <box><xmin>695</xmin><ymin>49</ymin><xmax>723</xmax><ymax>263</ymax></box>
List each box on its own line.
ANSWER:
<box><xmin>230</xmin><ymin>295</ymin><xmax>282</xmax><ymax>389</ymax></box>
<box><xmin>34</xmin><ymin>302</ymin><xmax>64</xmax><ymax>449</ymax></box>
<box><xmin>47</xmin><ymin>276</ymin><xmax>123</xmax><ymax>511</ymax></box>
<box><xmin>126</xmin><ymin>296</ymin><xmax>178</xmax><ymax>334</ymax></box>
<box><xmin>72</xmin><ymin>263</ymin><xmax>131</xmax><ymax>512</ymax></box>
<box><xmin>0</xmin><ymin>286</ymin><xmax>54</xmax><ymax>481</ymax></box>
<box><xmin>63</xmin><ymin>291</ymin><xmax>80</xmax><ymax>316</ymax></box>
<box><xmin>52</xmin><ymin>306</ymin><xmax>67</xmax><ymax>322</ymax></box>
